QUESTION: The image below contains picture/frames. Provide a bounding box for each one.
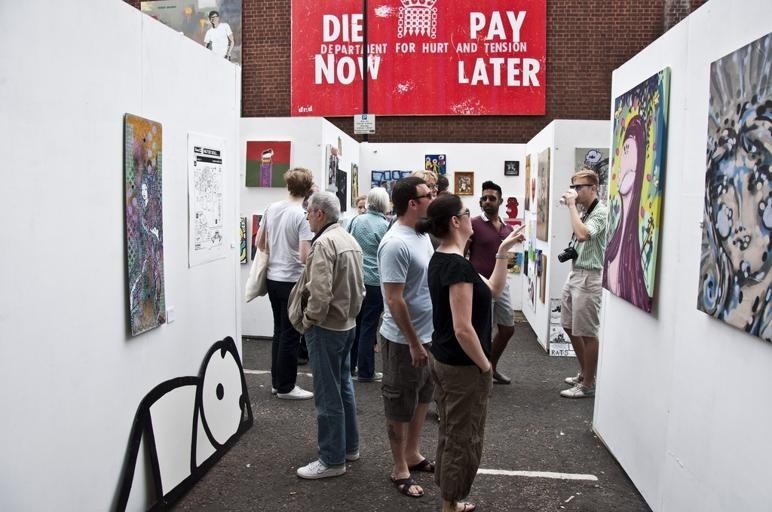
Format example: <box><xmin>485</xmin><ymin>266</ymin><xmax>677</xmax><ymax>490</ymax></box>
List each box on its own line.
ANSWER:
<box><xmin>503</xmin><ymin>159</ymin><xmax>521</xmax><ymax>176</ymax></box>
<box><xmin>453</xmin><ymin>169</ymin><xmax>476</xmax><ymax>197</ymax></box>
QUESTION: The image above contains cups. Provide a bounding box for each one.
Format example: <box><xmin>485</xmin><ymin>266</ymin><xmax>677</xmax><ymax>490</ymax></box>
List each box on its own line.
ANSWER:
<box><xmin>559</xmin><ymin>188</ymin><xmax>580</xmax><ymax>206</ymax></box>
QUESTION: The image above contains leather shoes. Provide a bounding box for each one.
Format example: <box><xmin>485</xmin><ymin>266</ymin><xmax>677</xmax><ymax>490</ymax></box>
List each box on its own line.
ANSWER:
<box><xmin>493</xmin><ymin>371</ymin><xmax>510</xmax><ymax>383</ymax></box>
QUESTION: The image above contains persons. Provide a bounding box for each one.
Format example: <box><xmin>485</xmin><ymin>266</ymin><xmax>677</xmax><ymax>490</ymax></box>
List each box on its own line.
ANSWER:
<box><xmin>204</xmin><ymin>11</ymin><xmax>235</xmax><ymax>62</ymax></box>
<box><xmin>347</xmin><ymin>170</ymin><xmax>513</xmax><ymax>498</ymax></box>
<box><xmin>256</xmin><ymin>167</ymin><xmax>366</xmax><ymax>479</ymax></box>
<box><xmin>413</xmin><ymin>191</ymin><xmax>525</xmax><ymax>511</ymax></box>
<box><xmin>557</xmin><ymin>171</ymin><xmax>606</xmax><ymax>399</ymax></box>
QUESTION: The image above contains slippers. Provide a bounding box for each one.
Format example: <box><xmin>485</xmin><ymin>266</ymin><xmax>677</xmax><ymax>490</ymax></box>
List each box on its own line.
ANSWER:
<box><xmin>458</xmin><ymin>501</ymin><xmax>476</xmax><ymax>512</ymax></box>
<box><xmin>409</xmin><ymin>458</ymin><xmax>435</xmax><ymax>472</ymax></box>
<box><xmin>389</xmin><ymin>474</ymin><xmax>424</xmax><ymax>497</ymax></box>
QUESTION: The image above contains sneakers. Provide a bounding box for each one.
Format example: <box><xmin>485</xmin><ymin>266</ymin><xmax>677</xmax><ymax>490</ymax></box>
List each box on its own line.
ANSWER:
<box><xmin>559</xmin><ymin>381</ymin><xmax>594</xmax><ymax>398</ymax></box>
<box><xmin>358</xmin><ymin>372</ymin><xmax>383</xmax><ymax>382</ymax></box>
<box><xmin>297</xmin><ymin>358</ymin><xmax>308</xmax><ymax>365</ymax></box>
<box><xmin>277</xmin><ymin>383</ymin><xmax>314</xmax><ymax>400</ymax></box>
<box><xmin>317</xmin><ymin>445</ymin><xmax>360</xmax><ymax>461</ymax></box>
<box><xmin>565</xmin><ymin>372</ymin><xmax>583</xmax><ymax>386</ymax></box>
<box><xmin>296</xmin><ymin>460</ymin><xmax>347</xmax><ymax>479</ymax></box>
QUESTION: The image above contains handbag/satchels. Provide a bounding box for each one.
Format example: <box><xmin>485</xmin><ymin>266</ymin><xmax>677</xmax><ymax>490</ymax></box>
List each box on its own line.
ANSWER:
<box><xmin>246</xmin><ymin>204</ymin><xmax>267</xmax><ymax>303</ymax></box>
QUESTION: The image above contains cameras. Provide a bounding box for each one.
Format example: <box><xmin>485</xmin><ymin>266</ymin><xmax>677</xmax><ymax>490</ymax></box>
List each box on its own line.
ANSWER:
<box><xmin>558</xmin><ymin>247</ymin><xmax>578</xmax><ymax>262</ymax></box>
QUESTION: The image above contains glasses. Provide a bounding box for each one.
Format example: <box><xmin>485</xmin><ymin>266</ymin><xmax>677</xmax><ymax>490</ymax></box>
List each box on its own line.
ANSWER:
<box><xmin>570</xmin><ymin>184</ymin><xmax>592</xmax><ymax>190</ymax></box>
<box><xmin>416</xmin><ymin>191</ymin><xmax>431</xmax><ymax>199</ymax></box>
<box><xmin>427</xmin><ymin>183</ymin><xmax>439</xmax><ymax>190</ymax></box>
<box><xmin>304</xmin><ymin>210</ymin><xmax>315</xmax><ymax>218</ymax></box>
<box><xmin>458</xmin><ymin>209</ymin><xmax>470</xmax><ymax>218</ymax></box>
<box><xmin>482</xmin><ymin>195</ymin><xmax>497</xmax><ymax>201</ymax></box>
